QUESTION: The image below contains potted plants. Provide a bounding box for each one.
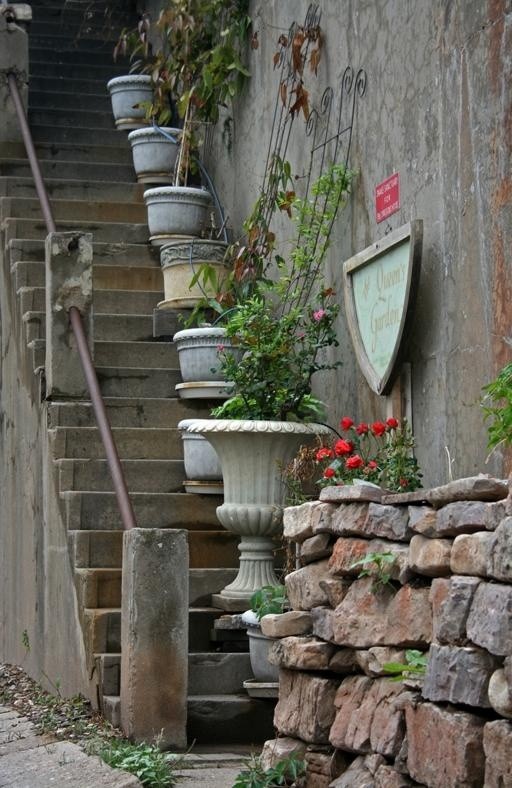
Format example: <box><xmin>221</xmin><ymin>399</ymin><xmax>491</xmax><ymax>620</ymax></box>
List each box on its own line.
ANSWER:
<box><xmin>241</xmin><ymin>585</ymin><xmax>291</xmax><ymax>684</ymax></box>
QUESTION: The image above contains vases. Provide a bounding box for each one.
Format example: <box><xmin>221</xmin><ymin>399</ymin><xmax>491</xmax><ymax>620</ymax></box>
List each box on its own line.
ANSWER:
<box><xmin>107</xmin><ymin>74</ymin><xmax>166</xmax><ymax>130</ymax></box>
<box><xmin>157</xmin><ymin>240</ymin><xmax>235</xmax><ymax>310</ymax></box>
<box><xmin>128</xmin><ymin>127</ymin><xmax>185</xmax><ymax>184</ymax></box>
<box><xmin>178</xmin><ymin>417</ymin><xmax>226</xmax><ymax>496</ymax></box>
<box><xmin>178</xmin><ymin>417</ymin><xmax>333</xmax><ymax>600</ymax></box>
<box><xmin>143</xmin><ymin>187</ymin><xmax>214</xmax><ymax>247</ymax></box>
<box><xmin>174</xmin><ymin>330</ymin><xmax>245</xmax><ymax>399</ymax></box>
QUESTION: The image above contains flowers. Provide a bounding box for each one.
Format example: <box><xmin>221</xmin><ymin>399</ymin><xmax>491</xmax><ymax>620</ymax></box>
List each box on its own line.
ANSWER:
<box><xmin>215</xmin><ymin>288</ymin><xmax>337</xmax><ymax>421</ymax></box>
